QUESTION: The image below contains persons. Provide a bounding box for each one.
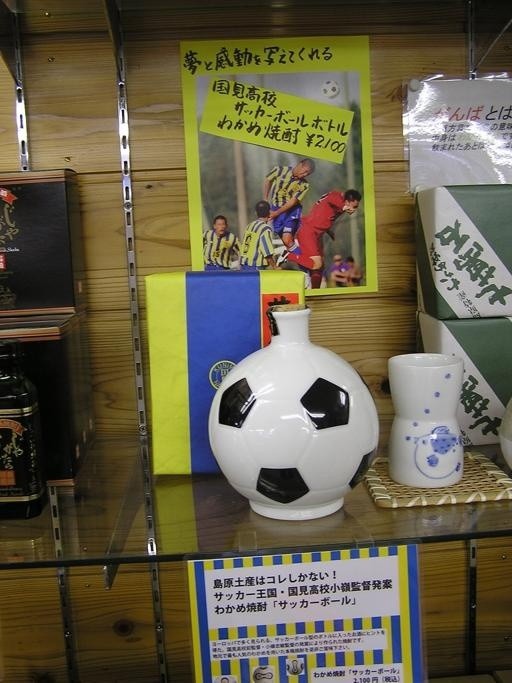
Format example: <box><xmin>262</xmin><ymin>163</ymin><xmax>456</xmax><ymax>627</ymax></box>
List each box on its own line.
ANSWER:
<box><xmin>276</xmin><ymin>190</ymin><xmax>362</xmax><ymax>289</ymax></box>
<box><xmin>263</xmin><ymin>159</ymin><xmax>316</xmax><ymax>255</ymax></box>
<box><xmin>239</xmin><ymin>201</ymin><xmax>281</xmax><ymax>271</ymax></box>
<box><xmin>323</xmin><ymin>254</ymin><xmax>363</xmax><ymax>287</ymax></box>
<box><xmin>202</xmin><ymin>215</ymin><xmax>240</xmax><ymax>270</ymax></box>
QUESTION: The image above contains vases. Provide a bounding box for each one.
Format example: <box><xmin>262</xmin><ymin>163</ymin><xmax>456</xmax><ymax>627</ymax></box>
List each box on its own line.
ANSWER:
<box><xmin>207</xmin><ymin>306</ymin><xmax>382</xmax><ymax>521</ymax></box>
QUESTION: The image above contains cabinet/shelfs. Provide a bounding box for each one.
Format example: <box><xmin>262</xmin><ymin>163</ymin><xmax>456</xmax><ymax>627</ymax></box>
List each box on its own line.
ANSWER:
<box><xmin>0</xmin><ymin>2</ymin><xmax>512</xmax><ymax>682</ymax></box>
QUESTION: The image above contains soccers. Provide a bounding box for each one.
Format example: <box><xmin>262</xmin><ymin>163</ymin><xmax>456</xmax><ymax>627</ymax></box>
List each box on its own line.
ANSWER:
<box><xmin>321</xmin><ymin>80</ymin><xmax>341</xmax><ymax>98</ymax></box>
